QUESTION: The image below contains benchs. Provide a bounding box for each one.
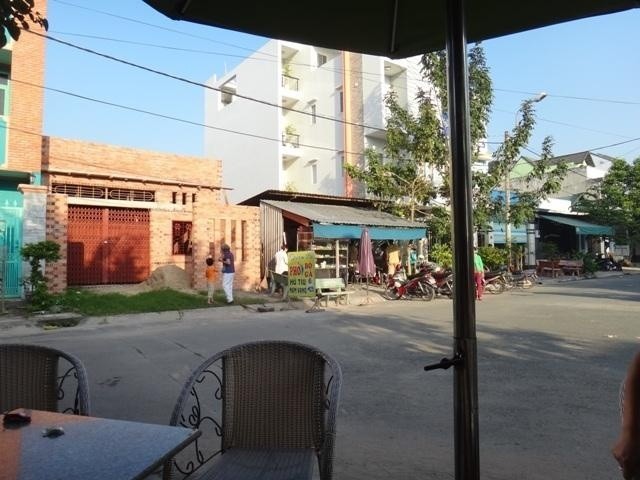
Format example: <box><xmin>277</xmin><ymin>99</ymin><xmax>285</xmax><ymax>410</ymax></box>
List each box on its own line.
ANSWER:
<box><xmin>314</xmin><ymin>278</ymin><xmax>355</xmax><ymax>306</ymax></box>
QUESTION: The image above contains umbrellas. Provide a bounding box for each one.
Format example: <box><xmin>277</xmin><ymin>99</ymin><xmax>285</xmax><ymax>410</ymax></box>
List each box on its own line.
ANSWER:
<box><xmin>358</xmin><ymin>227</ymin><xmax>378</xmax><ymax>302</ymax></box>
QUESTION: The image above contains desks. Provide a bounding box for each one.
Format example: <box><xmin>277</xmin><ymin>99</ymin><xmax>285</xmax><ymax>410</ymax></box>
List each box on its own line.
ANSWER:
<box><xmin>0</xmin><ymin>406</ymin><xmax>202</xmax><ymax>480</ymax></box>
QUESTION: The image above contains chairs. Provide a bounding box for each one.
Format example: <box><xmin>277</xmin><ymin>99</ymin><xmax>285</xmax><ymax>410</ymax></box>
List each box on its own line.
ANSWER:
<box><xmin>0</xmin><ymin>342</ymin><xmax>93</xmax><ymax>419</ymax></box>
<box><xmin>164</xmin><ymin>341</ymin><xmax>341</xmax><ymax>479</ymax></box>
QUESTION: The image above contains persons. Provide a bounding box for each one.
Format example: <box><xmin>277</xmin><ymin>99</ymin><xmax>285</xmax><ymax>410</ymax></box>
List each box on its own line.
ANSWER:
<box><xmin>610</xmin><ymin>350</ymin><xmax>640</xmax><ymax>480</ymax></box>
<box><xmin>205</xmin><ymin>258</ymin><xmax>219</xmax><ymax>305</ymax></box>
<box><xmin>274</xmin><ymin>245</ymin><xmax>289</xmax><ymax>299</ymax></box>
<box><xmin>474</xmin><ymin>246</ymin><xmax>486</xmax><ymax>301</ymax></box>
<box><xmin>219</xmin><ymin>243</ymin><xmax>236</xmax><ymax>304</ymax></box>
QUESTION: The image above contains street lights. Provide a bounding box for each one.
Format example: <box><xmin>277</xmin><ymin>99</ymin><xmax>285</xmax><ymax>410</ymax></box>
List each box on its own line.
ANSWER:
<box><xmin>504</xmin><ymin>91</ymin><xmax>548</xmax><ymax>271</ymax></box>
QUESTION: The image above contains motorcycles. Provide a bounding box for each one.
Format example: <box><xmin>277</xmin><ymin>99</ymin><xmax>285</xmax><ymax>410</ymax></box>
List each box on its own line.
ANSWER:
<box><xmin>386</xmin><ymin>263</ymin><xmax>540</xmax><ymax>301</ymax></box>
